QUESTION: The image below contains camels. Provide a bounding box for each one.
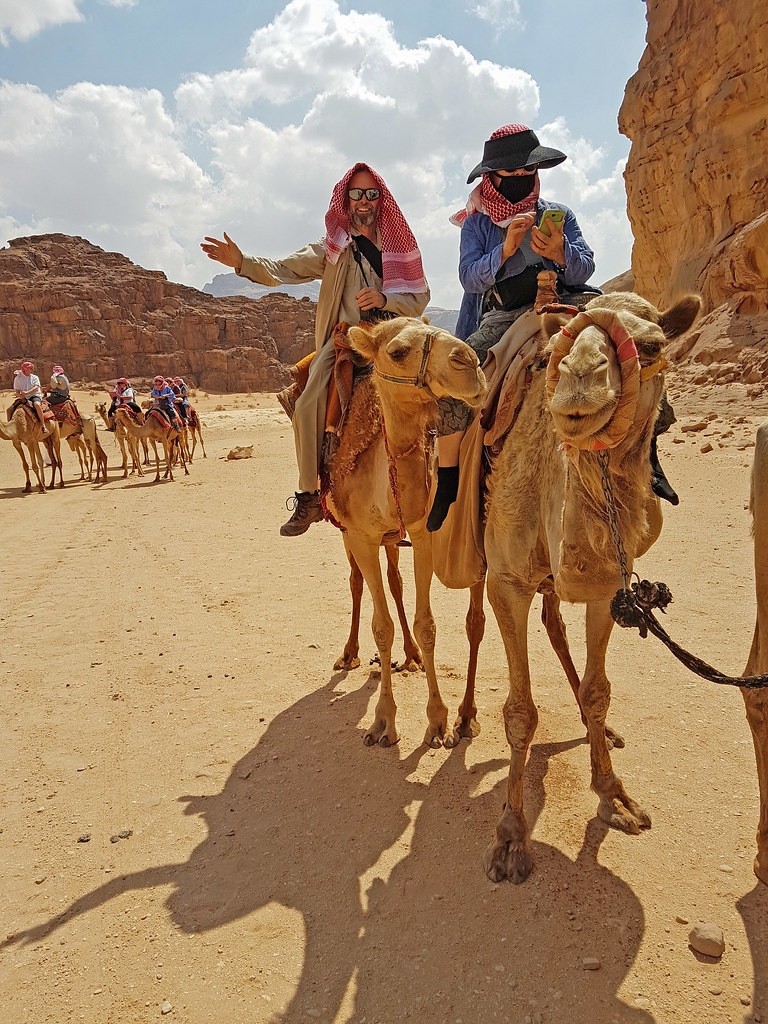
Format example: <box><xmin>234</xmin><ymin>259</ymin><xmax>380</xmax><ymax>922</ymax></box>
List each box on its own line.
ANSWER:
<box><xmin>741</xmin><ymin>424</ymin><xmax>768</xmax><ymax>888</ymax></box>
<box><xmin>0</xmin><ymin>399</ymin><xmax>108</xmax><ymax>494</ymax></box>
<box><xmin>276</xmin><ymin>291</ymin><xmax>703</xmax><ymax>886</ymax></box>
<box><xmin>95</xmin><ymin>396</ymin><xmax>207</xmax><ymax>482</ymax></box>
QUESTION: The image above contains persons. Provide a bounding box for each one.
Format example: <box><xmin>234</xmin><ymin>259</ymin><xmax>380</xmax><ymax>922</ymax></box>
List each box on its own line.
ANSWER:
<box><xmin>105</xmin><ymin>377</ymin><xmax>142</xmax><ymax>431</ymax></box>
<box><xmin>201</xmin><ymin>162</ymin><xmax>432</xmax><ymax>538</ymax></box>
<box><xmin>46</xmin><ymin>366</ymin><xmax>70</xmax><ymax>405</ymax></box>
<box><xmin>144</xmin><ymin>376</ymin><xmax>190</xmax><ymax>433</ymax></box>
<box><xmin>6</xmin><ymin>361</ymin><xmax>50</xmax><ymax>434</ymax></box>
<box><xmin>426</xmin><ymin>125</ymin><xmax>679</xmax><ymax>533</ymax></box>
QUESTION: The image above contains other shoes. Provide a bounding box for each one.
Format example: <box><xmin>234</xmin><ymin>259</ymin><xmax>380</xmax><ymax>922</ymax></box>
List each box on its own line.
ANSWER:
<box><xmin>176</xmin><ymin>425</ymin><xmax>181</xmax><ymax>433</ymax></box>
<box><xmin>42</xmin><ymin>426</ymin><xmax>50</xmax><ymax>435</ymax></box>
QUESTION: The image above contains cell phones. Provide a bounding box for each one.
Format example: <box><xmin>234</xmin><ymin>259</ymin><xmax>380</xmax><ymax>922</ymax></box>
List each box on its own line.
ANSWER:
<box><xmin>538</xmin><ymin>208</ymin><xmax>567</xmax><ymax>238</ymax></box>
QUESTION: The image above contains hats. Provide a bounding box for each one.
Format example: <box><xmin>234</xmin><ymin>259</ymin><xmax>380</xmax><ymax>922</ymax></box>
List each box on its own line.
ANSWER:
<box><xmin>466</xmin><ymin>129</ymin><xmax>568</xmax><ymax>185</ymax></box>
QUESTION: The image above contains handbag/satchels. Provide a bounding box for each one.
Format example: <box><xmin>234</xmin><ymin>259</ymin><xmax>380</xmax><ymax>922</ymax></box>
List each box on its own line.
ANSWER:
<box><xmin>489</xmin><ymin>262</ymin><xmax>564</xmax><ymax>312</ymax></box>
<box><xmin>359</xmin><ymin>303</ymin><xmax>398</xmax><ymax>325</ymax></box>
<box><xmin>159</xmin><ymin>399</ymin><xmax>168</xmax><ymax>410</ymax></box>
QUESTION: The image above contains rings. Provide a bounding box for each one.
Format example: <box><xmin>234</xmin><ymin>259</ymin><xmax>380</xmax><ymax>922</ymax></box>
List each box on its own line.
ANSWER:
<box><xmin>541</xmin><ymin>245</ymin><xmax>546</xmax><ymax>251</ymax></box>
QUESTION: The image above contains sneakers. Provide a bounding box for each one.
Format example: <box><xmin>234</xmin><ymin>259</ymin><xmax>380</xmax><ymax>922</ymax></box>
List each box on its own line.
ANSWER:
<box><xmin>279</xmin><ymin>489</ymin><xmax>324</xmax><ymax>536</ymax></box>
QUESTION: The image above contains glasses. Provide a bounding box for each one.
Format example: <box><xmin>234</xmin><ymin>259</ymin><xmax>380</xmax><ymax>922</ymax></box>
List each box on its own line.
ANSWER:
<box><xmin>346</xmin><ymin>187</ymin><xmax>381</xmax><ymax>201</ymax></box>
<box><xmin>500</xmin><ymin>162</ymin><xmax>539</xmax><ymax>173</ymax></box>
